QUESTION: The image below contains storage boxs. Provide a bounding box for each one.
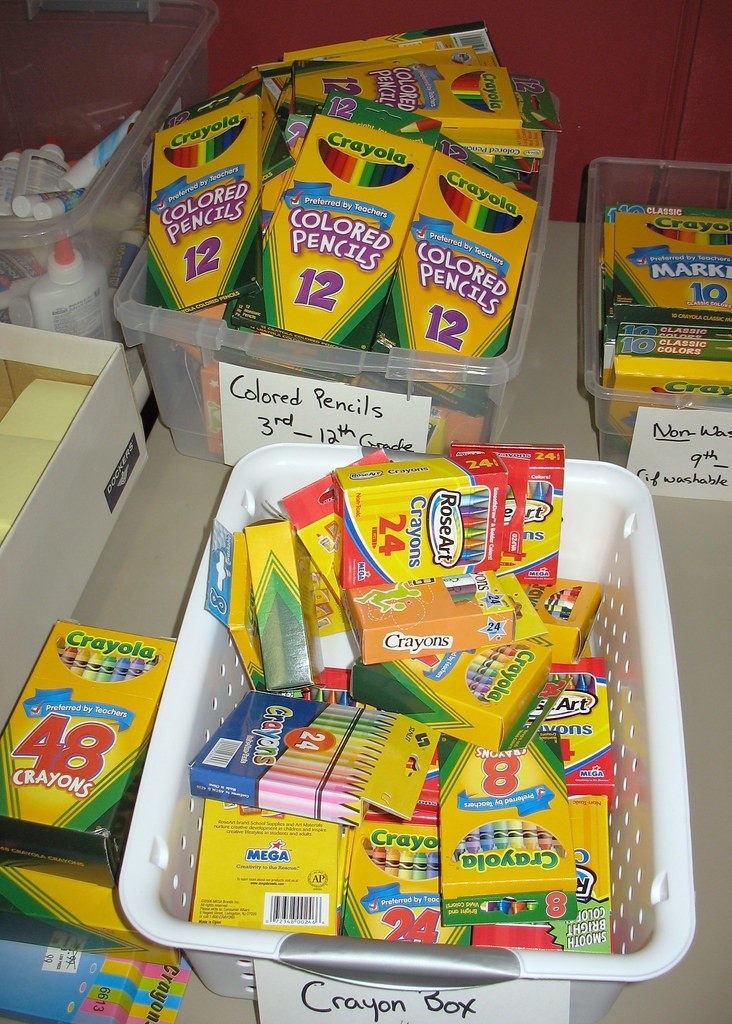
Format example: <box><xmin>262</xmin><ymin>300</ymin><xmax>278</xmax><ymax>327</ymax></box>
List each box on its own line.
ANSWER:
<box><xmin>146</xmin><ymin>19</ymin><xmax>564</xmax><ymax>466</ymax></box>
<box><xmin>0</xmin><ymin>322</ymin><xmax>150</xmax><ymax>737</ymax></box>
<box><xmin>584</xmin><ymin>156</ymin><xmax>732</xmax><ymax>502</ymax></box>
<box><xmin>119</xmin><ymin>442</ymin><xmax>696</xmax><ymax>1001</ymax></box>
<box><xmin>0</xmin><ymin>617</ymin><xmax>194</xmax><ymax>1024</ymax></box>
<box><xmin>113</xmin><ymin>90</ymin><xmax>557</xmax><ymax>464</ymax></box>
<box><xmin>191</xmin><ymin>443</ymin><xmax>657</xmax><ymax>955</ymax></box>
<box><xmin>1</xmin><ymin>0</ymin><xmax>219</xmax><ymax>443</ymax></box>
<box><xmin>598</xmin><ymin>203</ymin><xmax>732</xmax><ymax>452</ymax></box>
